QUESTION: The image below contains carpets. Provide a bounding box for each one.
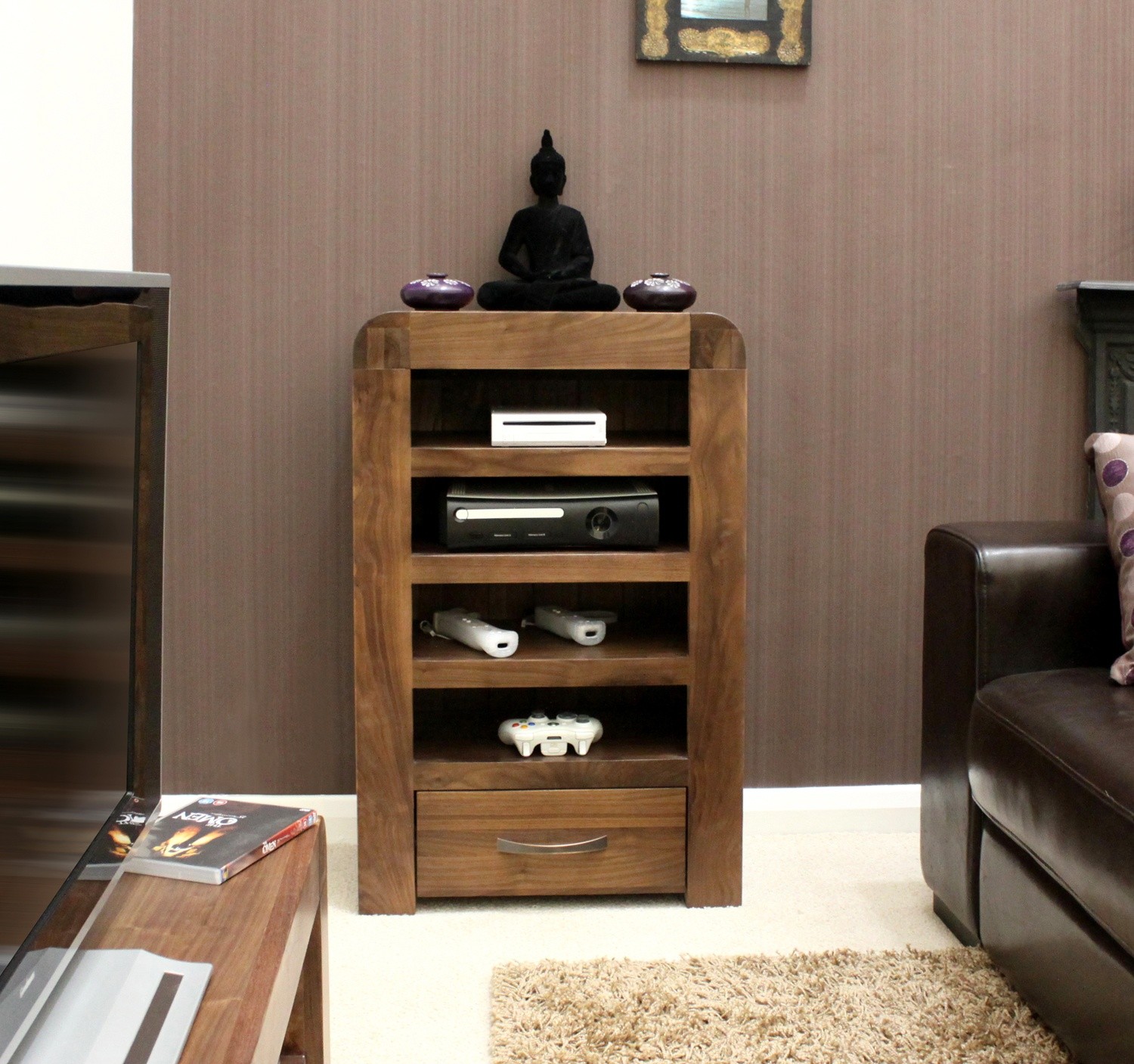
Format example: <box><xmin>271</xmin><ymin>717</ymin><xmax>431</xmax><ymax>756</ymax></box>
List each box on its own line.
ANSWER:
<box><xmin>487</xmin><ymin>947</ymin><xmax>1075</xmax><ymax>1063</ymax></box>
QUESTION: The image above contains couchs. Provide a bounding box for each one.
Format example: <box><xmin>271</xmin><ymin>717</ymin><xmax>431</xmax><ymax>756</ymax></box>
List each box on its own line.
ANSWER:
<box><xmin>920</xmin><ymin>518</ymin><xmax>1133</xmax><ymax>1063</ymax></box>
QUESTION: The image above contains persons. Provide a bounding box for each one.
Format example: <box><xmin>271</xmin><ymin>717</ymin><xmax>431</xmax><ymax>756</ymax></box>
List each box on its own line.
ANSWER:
<box><xmin>477</xmin><ymin>129</ymin><xmax>621</xmax><ymax>312</ymax></box>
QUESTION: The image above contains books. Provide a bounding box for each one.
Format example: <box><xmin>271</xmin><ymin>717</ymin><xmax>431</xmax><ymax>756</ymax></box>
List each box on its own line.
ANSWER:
<box><xmin>78</xmin><ymin>796</ymin><xmax>159</xmax><ymax>881</ymax></box>
<box><xmin>126</xmin><ymin>798</ymin><xmax>318</xmax><ymax>884</ymax></box>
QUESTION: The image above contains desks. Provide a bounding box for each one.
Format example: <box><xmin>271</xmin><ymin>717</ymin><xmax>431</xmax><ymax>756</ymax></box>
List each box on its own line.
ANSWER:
<box><xmin>0</xmin><ymin>814</ymin><xmax>329</xmax><ymax>1063</ymax></box>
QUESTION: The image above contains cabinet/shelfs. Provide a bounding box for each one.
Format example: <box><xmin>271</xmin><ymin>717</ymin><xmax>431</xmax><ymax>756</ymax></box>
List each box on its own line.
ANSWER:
<box><xmin>347</xmin><ymin>312</ymin><xmax>741</xmax><ymax>908</ymax></box>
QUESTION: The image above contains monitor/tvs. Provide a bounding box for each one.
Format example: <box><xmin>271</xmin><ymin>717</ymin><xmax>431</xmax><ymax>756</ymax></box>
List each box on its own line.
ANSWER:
<box><xmin>0</xmin><ymin>265</ymin><xmax>172</xmax><ymax>1064</ymax></box>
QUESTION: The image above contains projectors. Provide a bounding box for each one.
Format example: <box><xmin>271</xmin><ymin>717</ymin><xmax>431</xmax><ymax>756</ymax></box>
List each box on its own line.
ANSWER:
<box><xmin>446</xmin><ymin>484</ymin><xmax>660</xmax><ymax>549</ymax></box>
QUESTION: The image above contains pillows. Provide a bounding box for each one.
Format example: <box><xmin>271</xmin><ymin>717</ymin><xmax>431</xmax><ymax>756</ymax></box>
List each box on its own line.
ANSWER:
<box><xmin>1086</xmin><ymin>431</ymin><xmax>1133</xmax><ymax>682</ymax></box>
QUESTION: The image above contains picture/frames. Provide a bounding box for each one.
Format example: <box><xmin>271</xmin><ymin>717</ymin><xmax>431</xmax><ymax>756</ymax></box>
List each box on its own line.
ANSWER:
<box><xmin>636</xmin><ymin>0</ymin><xmax>810</xmax><ymax>65</ymax></box>
